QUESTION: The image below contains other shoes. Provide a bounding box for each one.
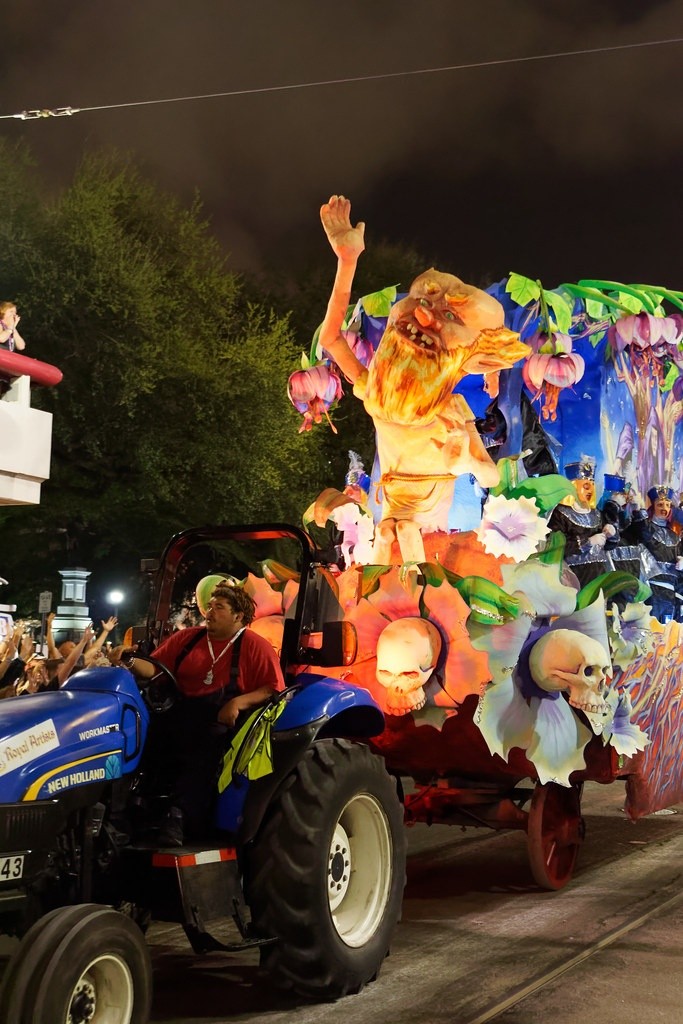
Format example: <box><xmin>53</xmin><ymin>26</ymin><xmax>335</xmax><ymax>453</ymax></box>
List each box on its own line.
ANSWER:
<box><xmin>160</xmin><ymin>814</ymin><xmax>183</xmax><ymax>847</ymax></box>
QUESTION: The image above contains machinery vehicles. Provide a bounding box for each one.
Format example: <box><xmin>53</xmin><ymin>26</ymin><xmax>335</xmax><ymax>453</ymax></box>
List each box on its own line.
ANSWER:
<box><xmin>0</xmin><ymin>525</ymin><xmax>408</xmax><ymax>1024</ymax></box>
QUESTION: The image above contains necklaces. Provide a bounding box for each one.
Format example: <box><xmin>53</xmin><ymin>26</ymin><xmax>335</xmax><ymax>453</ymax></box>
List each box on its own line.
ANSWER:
<box><xmin>203</xmin><ymin>627</ymin><xmax>246</xmax><ymax>685</ymax></box>
<box><xmin>8</xmin><ymin>339</ymin><xmax>14</xmax><ymax>352</ymax></box>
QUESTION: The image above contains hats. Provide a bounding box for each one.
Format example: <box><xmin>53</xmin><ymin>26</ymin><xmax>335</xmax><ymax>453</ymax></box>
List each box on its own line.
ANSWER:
<box><xmin>564</xmin><ymin>462</ymin><xmax>596</xmax><ymax>481</ymax></box>
<box><xmin>603</xmin><ymin>473</ymin><xmax>626</xmax><ymax>493</ymax></box>
<box><xmin>647</xmin><ymin>486</ymin><xmax>673</xmax><ymax>501</ymax></box>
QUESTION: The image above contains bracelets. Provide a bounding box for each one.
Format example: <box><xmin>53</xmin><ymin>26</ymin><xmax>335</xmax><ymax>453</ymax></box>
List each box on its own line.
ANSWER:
<box><xmin>2</xmin><ymin>639</ymin><xmax>9</xmax><ymax>642</ymax></box>
<box><xmin>126</xmin><ymin>657</ymin><xmax>133</xmax><ymax>664</ymax></box>
<box><xmin>127</xmin><ymin>658</ymin><xmax>136</xmax><ymax>669</ymax></box>
<box><xmin>6</xmin><ymin>329</ymin><xmax>12</xmax><ymax>333</ymax></box>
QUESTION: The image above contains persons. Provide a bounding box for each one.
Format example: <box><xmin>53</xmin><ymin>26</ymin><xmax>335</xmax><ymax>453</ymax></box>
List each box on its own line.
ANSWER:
<box><xmin>546</xmin><ymin>462</ymin><xmax>683</xmax><ymax>623</ymax></box>
<box><xmin>0</xmin><ymin>592</ymin><xmax>203</xmax><ymax>699</ymax></box>
<box><xmin>318</xmin><ymin>196</ymin><xmax>531</xmax><ymax>575</ymax></box>
<box><xmin>0</xmin><ymin>302</ymin><xmax>25</xmax><ymax>400</ymax></box>
<box><xmin>111</xmin><ymin>583</ymin><xmax>286</xmax><ymax>849</ymax></box>
<box><xmin>474</xmin><ymin>370</ymin><xmax>558</xmax><ymax>478</ymax></box>
<box><xmin>345</xmin><ymin>450</ymin><xmax>370</xmax><ymax>494</ymax></box>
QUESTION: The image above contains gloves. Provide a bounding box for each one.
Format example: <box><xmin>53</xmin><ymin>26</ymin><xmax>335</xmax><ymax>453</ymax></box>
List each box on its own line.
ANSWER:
<box><xmin>588</xmin><ymin>533</ymin><xmax>606</xmax><ymax>546</ymax></box>
<box><xmin>602</xmin><ymin>524</ymin><xmax>616</xmax><ymax>538</ymax></box>
<box><xmin>675</xmin><ymin>556</ymin><xmax>683</xmax><ymax>570</ymax></box>
